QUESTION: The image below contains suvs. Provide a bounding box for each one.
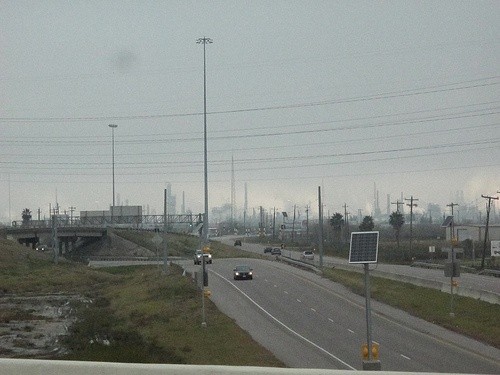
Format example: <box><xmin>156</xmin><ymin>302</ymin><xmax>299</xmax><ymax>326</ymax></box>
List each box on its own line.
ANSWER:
<box><xmin>193</xmin><ymin>249</ymin><xmax>212</xmax><ymax>265</ymax></box>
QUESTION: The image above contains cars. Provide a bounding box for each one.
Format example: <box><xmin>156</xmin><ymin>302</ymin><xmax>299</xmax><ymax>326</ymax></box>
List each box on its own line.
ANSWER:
<box><xmin>299</xmin><ymin>250</ymin><xmax>315</xmax><ymax>261</ymax></box>
<box><xmin>272</xmin><ymin>247</ymin><xmax>281</xmax><ymax>255</ymax></box>
<box><xmin>234</xmin><ymin>239</ymin><xmax>242</xmax><ymax>246</ymax></box>
<box><xmin>232</xmin><ymin>264</ymin><xmax>254</xmax><ymax>280</ymax></box>
<box><xmin>263</xmin><ymin>246</ymin><xmax>272</xmax><ymax>253</ymax></box>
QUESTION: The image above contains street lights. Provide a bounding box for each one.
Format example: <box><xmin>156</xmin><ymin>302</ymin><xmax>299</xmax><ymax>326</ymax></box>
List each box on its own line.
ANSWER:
<box><xmin>196</xmin><ymin>35</ymin><xmax>214</xmax><ymax>286</ymax></box>
<box><xmin>108</xmin><ymin>124</ymin><xmax>118</xmax><ymax>224</ymax></box>
